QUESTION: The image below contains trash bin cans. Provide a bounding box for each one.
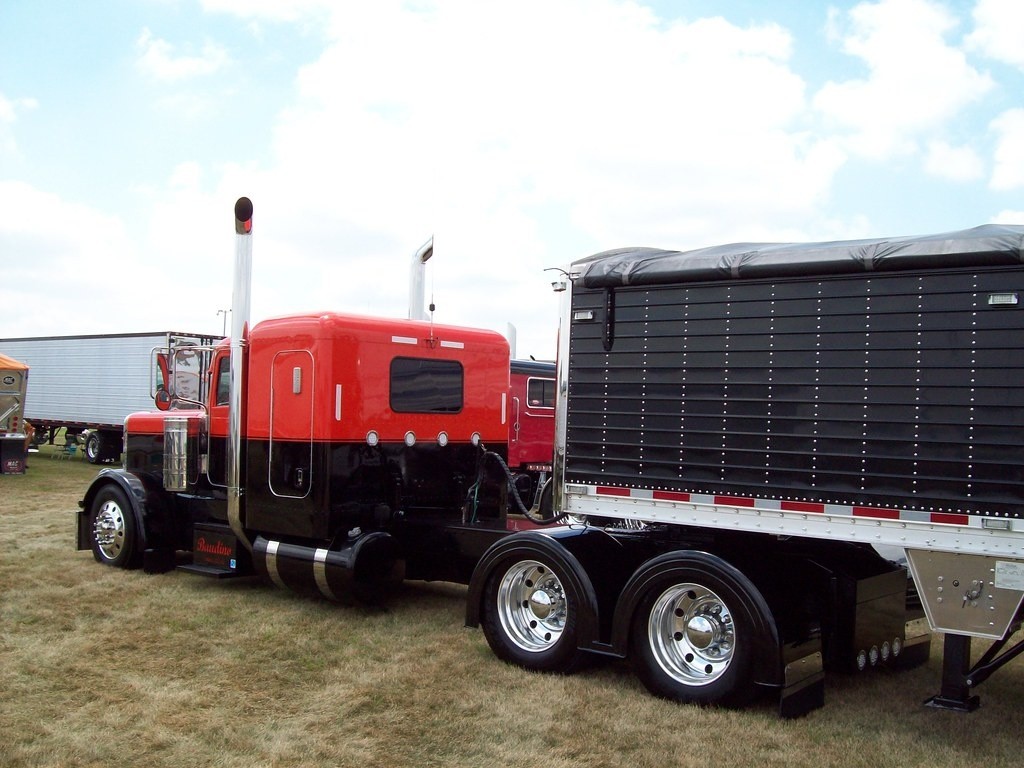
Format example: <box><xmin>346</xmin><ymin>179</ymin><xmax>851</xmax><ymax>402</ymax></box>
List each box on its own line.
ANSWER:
<box><xmin>0</xmin><ymin>433</ymin><xmax>29</xmax><ymax>475</ymax></box>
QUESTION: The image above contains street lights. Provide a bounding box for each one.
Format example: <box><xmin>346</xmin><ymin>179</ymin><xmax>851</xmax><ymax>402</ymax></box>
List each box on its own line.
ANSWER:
<box><xmin>215</xmin><ymin>307</ymin><xmax>232</xmax><ymax>337</ymax></box>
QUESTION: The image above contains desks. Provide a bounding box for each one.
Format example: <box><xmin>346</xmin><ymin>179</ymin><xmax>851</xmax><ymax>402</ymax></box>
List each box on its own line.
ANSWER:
<box><xmin>46</xmin><ymin>444</ymin><xmax>69</xmax><ymax>459</ymax></box>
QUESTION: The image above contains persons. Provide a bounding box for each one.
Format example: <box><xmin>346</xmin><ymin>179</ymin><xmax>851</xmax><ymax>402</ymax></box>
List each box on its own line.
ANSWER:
<box><xmin>65</xmin><ymin>427</ymin><xmax>88</xmax><ymax>446</ymax></box>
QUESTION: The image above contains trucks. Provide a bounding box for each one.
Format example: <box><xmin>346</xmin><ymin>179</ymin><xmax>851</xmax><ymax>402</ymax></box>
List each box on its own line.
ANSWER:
<box><xmin>72</xmin><ymin>196</ymin><xmax>1023</xmax><ymax>714</ymax></box>
<box><xmin>0</xmin><ymin>331</ymin><xmax>228</xmax><ymax>463</ymax></box>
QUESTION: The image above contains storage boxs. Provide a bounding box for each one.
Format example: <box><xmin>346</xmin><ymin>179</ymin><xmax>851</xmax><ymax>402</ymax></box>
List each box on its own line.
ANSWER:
<box><xmin>0</xmin><ymin>369</ymin><xmax>28</xmax><ymax>433</ymax></box>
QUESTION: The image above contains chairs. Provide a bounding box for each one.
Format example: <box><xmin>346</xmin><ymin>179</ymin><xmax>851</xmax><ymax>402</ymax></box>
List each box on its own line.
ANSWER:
<box><xmin>80</xmin><ymin>444</ymin><xmax>86</xmax><ymax>462</ymax></box>
<box><xmin>61</xmin><ymin>447</ymin><xmax>76</xmax><ymax>460</ymax></box>
